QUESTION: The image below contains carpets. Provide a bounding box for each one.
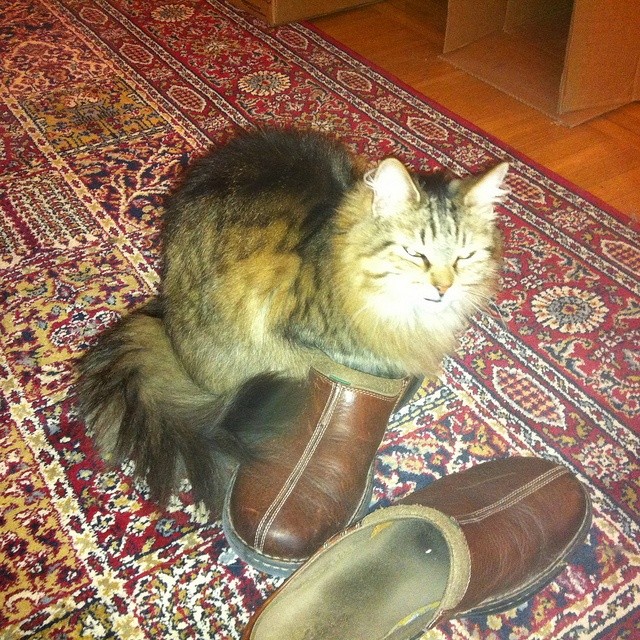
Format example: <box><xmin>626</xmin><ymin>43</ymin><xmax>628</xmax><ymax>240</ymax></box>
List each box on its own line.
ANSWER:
<box><xmin>0</xmin><ymin>0</ymin><xmax>640</xmax><ymax>640</ymax></box>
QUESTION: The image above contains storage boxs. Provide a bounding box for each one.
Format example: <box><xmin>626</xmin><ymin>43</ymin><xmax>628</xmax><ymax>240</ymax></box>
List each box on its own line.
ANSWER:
<box><xmin>435</xmin><ymin>0</ymin><xmax>639</xmax><ymax>131</ymax></box>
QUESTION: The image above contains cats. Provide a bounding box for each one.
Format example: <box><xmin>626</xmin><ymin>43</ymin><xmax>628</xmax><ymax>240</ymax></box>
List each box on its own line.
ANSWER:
<box><xmin>69</xmin><ymin>125</ymin><xmax>511</xmax><ymax>517</ymax></box>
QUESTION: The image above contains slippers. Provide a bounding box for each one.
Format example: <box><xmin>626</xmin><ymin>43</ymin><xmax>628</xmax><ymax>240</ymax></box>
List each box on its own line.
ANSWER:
<box><xmin>241</xmin><ymin>457</ymin><xmax>592</xmax><ymax>640</ymax></box>
<box><xmin>223</xmin><ymin>360</ymin><xmax>416</xmax><ymax>578</ymax></box>
